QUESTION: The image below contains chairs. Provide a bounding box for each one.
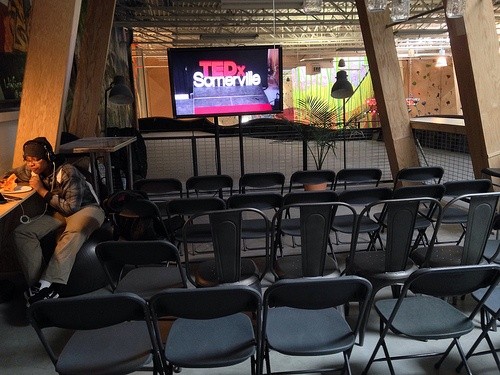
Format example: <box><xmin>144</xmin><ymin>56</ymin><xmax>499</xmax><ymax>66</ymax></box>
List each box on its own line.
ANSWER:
<box><xmin>28</xmin><ymin>167</ymin><xmax>500</xmax><ymax>375</ymax></box>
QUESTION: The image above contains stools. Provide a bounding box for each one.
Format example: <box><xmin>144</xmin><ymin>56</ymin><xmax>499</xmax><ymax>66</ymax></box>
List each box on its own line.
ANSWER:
<box><xmin>42</xmin><ymin>219</ymin><xmax>115</xmax><ymax>296</ymax></box>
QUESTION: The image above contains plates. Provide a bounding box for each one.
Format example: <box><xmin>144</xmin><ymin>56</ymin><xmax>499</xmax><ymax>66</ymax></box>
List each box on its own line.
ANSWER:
<box><xmin>0</xmin><ymin>186</ymin><xmax>32</xmax><ymax>194</ymax></box>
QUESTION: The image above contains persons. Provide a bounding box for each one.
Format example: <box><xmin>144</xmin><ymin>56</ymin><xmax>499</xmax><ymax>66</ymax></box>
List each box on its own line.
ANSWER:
<box><xmin>4</xmin><ymin>137</ymin><xmax>106</xmax><ymax>306</ymax></box>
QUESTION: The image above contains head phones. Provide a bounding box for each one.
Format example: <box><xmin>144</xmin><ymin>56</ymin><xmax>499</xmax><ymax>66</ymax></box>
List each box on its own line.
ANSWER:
<box><xmin>22</xmin><ymin>140</ymin><xmax>56</xmax><ymax>163</ymax></box>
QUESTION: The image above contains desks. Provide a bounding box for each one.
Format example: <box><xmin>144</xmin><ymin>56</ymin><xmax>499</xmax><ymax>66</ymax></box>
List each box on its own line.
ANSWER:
<box><xmin>0</xmin><ymin>181</ymin><xmax>36</xmax><ymax>221</ymax></box>
<box><xmin>60</xmin><ymin>136</ymin><xmax>138</xmax><ymax>199</ymax></box>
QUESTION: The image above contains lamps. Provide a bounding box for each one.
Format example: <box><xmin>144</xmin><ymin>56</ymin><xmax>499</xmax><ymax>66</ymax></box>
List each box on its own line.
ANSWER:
<box><xmin>220</xmin><ymin>0</ymin><xmax>304</xmax><ymax>10</ymax></box>
<box><xmin>104</xmin><ymin>75</ymin><xmax>133</xmax><ymax>135</ymax></box>
<box><xmin>331</xmin><ymin>60</ymin><xmax>354</xmax><ymax>189</ymax></box>
<box><xmin>200</xmin><ymin>32</ymin><xmax>259</xmax><ymax>42</ymax></box>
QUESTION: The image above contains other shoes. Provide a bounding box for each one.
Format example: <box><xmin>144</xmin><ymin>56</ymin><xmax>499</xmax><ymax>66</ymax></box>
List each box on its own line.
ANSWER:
<box><xmin>39</xmin><ymin>287</ymin><xmax>59</xmax><ymax>301</ymax></box>
<box><xmin>23</xmin><ymin>281</ymin><xmax>41</xmax><ymax>312</ymax></box>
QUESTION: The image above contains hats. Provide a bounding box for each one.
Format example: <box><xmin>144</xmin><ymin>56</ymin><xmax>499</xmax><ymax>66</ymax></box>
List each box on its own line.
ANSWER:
<box><xmin>23</xmin><ymin>137</ymin><xmax>53</xmax><ymax>163</ymax></box>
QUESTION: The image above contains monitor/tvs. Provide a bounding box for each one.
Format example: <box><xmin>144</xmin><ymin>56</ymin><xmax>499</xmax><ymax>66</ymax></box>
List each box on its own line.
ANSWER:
<box><xmin>167</xmin><ymin>45</ymin><xmax>283</xmax><ymax>120</ymax></box>
